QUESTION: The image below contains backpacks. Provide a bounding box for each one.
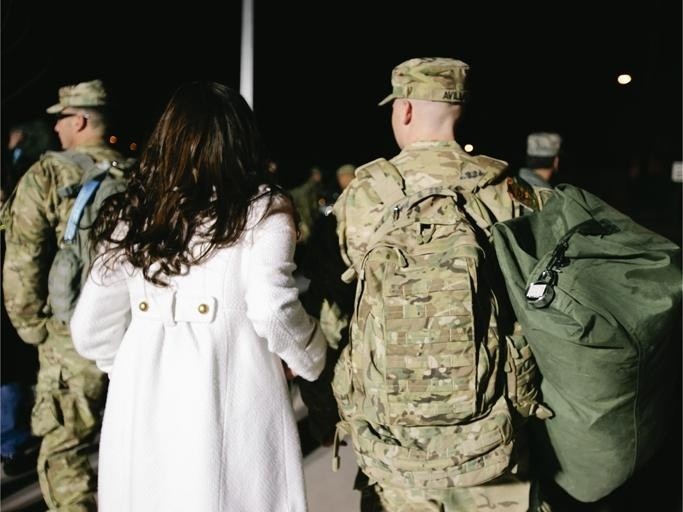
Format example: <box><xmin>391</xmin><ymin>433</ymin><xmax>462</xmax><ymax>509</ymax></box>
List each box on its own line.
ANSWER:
<box><xmin>40</xmin><ymin>150</ymin><xmax>138</xmax><ymax>327</ymax></box>
<box><xmin>331</xmin><ymin>157</ymin><xmax>554</xmax><ymax>491</ymax></box>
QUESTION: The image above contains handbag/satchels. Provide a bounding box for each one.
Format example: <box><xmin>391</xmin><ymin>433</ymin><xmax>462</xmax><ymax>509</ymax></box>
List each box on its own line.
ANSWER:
<box><xmin>489</xmin><ymin>182</ymin><xmax>683</xmax><ymax>502</ymax></box>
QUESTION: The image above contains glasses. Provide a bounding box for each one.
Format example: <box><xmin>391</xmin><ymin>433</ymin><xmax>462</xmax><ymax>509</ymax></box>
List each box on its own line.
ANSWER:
<box><xmin>53</xmin><ymin>113</ymin><xmax>76</xmax><ymax>126</ymax></box>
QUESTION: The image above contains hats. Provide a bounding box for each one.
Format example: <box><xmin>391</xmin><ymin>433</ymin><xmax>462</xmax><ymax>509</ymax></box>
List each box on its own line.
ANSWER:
<box><xmin>527</xmin><ymin>133</ymin><xmax>562</xmax><ymax>157</ymax></box>
<box><xmin>377</xmin><ymin>57</ymin><xmax>471</xmax><ymax>106</ymax></box>
<box><xmin>46</xmin><ymin>79</ymin><xmax>107</xmax><ymax>114</ymax></box>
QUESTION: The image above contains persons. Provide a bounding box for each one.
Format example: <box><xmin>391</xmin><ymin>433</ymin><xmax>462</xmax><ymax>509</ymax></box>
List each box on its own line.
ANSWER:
<box><xmin>0</xmin><ymin>80</ymin><xmax>129</xmax><ymax>512</ymax></box>
<box><xmin>293</xmin><ymin>57</ymin><xmax>557</xmax><ymax>512</ymax></box>
<box><xmin>518</xmin><ymin>132</ymin><xmax>562</xmax><ymax>188</ymax></box>
<box><xmin>70</xmin><ymin>73</ymin><xmax>338</xmax><ymax>512</ymax></box>
<box><xmin>287</xmin><ymin>163</ymin><xmax>356</xmax><ymax>237</ymax></box>
